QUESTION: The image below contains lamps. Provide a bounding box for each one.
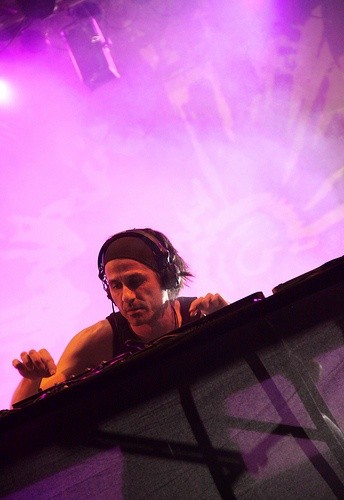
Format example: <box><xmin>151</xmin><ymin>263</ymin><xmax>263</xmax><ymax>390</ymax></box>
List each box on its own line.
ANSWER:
<box><xmin>43</xmin><ymin>0</ymin><xmax>120</xmax><ymax>95</ymax></box>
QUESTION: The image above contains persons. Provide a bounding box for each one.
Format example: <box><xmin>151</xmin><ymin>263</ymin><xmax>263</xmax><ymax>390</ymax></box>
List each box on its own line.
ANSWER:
<box><xmin>10</xmin><ymin>228</ymin><xmax>258</xmax><ymax>500</ymax></box>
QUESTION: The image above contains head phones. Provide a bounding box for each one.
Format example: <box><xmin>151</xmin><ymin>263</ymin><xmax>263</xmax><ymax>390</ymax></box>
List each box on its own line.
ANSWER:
<box><xmin>98</xmin><ymin>231</ymin><xmax>181</xmax><ymax>302</ymax></box>
